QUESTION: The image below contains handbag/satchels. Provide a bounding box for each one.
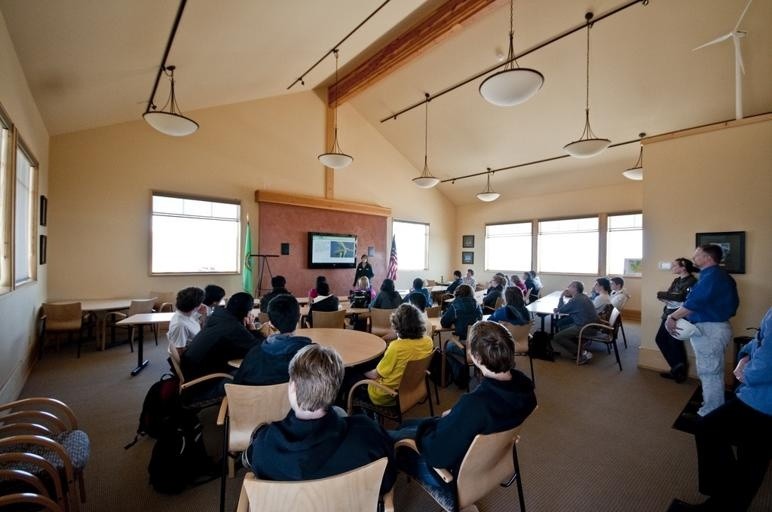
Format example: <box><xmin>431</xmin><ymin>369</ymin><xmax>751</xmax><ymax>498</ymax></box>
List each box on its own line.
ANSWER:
<box><xmin>428</xmin><ymin>346</ymin><xmax>455</xmax><ymax>388</ymax></box>
<box><xmin>145</xmin><ymin>423</ymin><xmax>227</xmax><ymax>497</ymax></box>
<box><xmin>348</xmin><ymin>288</ymin><xmax>372</xmax><ymax>309</ymax></box>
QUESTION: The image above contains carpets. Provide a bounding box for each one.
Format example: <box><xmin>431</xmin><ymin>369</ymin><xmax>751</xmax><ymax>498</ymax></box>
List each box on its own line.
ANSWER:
<box><xmin>669</xmin><ymin>384</ymin><xmax>734</xmax><ymax>437</ymax></box>
<box><xmin>664</xmin><ymin>497</ymin><xmax>706</xmax><ymax>512</ymax></box>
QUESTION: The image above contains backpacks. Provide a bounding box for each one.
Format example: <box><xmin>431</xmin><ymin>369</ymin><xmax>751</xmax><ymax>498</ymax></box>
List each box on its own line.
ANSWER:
<box><xmin>527</xmin><ymin>327</ymin><xmax>556</xmax><ymax>363</ymax></box>
<box><xmin>134</xmin><ymin>369</ymin><xmax>182</xmax><ymax>439</ymax></box>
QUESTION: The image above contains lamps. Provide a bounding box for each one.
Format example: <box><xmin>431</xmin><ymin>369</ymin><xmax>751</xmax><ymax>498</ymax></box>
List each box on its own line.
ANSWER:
<box><xmin>408</xmin><ymin>96</ymin><xmax>448</xmax><ymax>188</ymax></box>
<box><xmin>313</xmin><ymin>44</ymin><xmax>356</xmax><ymax>170</ymax></box>
<box><xmin>140</xmin><ymin>63</ymin><xmax>201</xmax><ymax>138</ymax></box>
<box><xmin>474</xmin><ymin>170</ymin><xmax>504</xmax><ymax>205</ymax></box>
<box><xmin>561</xmin><ymin>10</ymin><xmax>615</xmax><ymax>157</ymax></box>
<box><xmin>623</xmin><ymin>132</ymin><xmax>646</xmax><ymax>183</ymax></box>
<box><xmin>476</xmin><ymin>0</ymin><xmax>548</xmax><ymax>111</ymax></box>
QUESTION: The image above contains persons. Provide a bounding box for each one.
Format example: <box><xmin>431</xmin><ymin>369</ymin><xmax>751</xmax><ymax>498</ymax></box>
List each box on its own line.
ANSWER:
<box><xmin>242</xmin><ymin>344</ymin><xmax>398</xmax><ymax>512</ymax></box>
<box><xmin>350</xmin><ymin>269</ymin><xmax>630</xmax><ymax>365</ymax></box>
<box><xmin>695</xmin><ymin>306</ymin><xmax>772</xmax><ymax>512</ymax></box>
<box><xmin>168</xmin><ymin>276</ymin><xmax>339</xmax><ymax>406</ymax></box>
<box><xmin>665</xmin><ymin>243</ymin><xmax>739</xmax><ymax>418</ymax></box>
<box><xmin>655</xmin><ymin>258</ymin><xmax>698</xmax><ymax>384</ymax></box>
<box><xmin>338</xmin><ymin>302</ymin><xmax>434</xmax><ymax>408</ymax></box>
<box><xmin>385</xmin><ymin>320</ymin><xmax>537</xmax><ymax>497</ymax></box>
<box><xmin>352</xmin><ymin>255</ymin><xmax>374</xmax><ymax>289</ymax></box>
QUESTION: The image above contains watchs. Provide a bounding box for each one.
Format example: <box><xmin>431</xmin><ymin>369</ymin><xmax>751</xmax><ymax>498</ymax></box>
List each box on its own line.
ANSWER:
<box><xmin>667</xmin><ymin>314</ymin><xmax>678</xmax><ymax>321</ymax></box>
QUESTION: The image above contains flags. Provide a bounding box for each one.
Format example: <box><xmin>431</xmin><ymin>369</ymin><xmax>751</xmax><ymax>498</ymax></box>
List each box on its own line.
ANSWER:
<box><xmin>243</xmin><ymin>224</ymin><xmax>253</xmax><ymax>295</ymax></box>
<box><xmin>386</xmin><ymin>236</ymin><xmax>397</xmax><ymax>281</ymax></box>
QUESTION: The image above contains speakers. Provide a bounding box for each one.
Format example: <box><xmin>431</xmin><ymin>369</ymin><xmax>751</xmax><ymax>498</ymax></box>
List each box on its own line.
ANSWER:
<box><xmin>368</xmin><ymin>248</ymin><xmax>375</xmax><ymax>256</ymax></box>
<box><xmin>281</xmin><ymin>242</ymin><xmax>289</xmax><ymax>254</ymax></box>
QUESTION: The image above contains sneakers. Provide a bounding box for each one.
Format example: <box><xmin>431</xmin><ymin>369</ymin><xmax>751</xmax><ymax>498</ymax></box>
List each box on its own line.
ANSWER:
<box><xmin>241</xmin><ymin>421</ymin><xmax>268</xmax><ymax>470</ymax></box>
<box><xmin>659</xmin><ymin>369</ymin><xmax>679</xmax><ymax>380</ymax></box>
<box><xmin>674</xmin><ymin>363</ymin><xmax>686</xmax><ymax>385</ymax></box>
<box><xmin>571</xmin><ymin>350</ymin><xmax>593</xmax><ymax>366</ymax></box>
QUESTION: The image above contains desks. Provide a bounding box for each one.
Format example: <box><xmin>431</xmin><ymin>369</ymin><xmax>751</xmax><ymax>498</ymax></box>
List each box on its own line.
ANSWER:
<box><xmin>45</xmin><ymin>298</ymin><xmax>154</xmax><ymax>350</ymax></box>
<box><xmin>112</xmin><ymin>306</ymin><xmax>370</xmax><ymax>377</ymax></box>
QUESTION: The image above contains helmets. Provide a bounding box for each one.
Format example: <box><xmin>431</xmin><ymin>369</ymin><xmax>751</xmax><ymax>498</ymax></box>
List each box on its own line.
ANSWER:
<box><xmin>663</xmin><ymin>314</ymin><xmax>703</xmax><ymax>342</ymax></box>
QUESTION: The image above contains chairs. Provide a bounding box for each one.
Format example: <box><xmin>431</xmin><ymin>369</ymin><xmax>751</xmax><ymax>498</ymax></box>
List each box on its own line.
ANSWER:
<box><xmin>166</xmin><ymin>342</ymin><xmax>236</xmax><ymax>484</ymax></box>
<box><xmin>149</xmin><ymin>290</ymin><xmax>173</xmax><ymax>314</ymax></box>
<box><xmin>1</xmin><ymin>397</ymin><xmax>88</xmax><ymax>512</ymax></box>
<box><xmin>252</xmin><ymin>281</ymin><xmax>632</xmax><ymax>430</ymax></box>
<box><xmin>215</xmin><ymin>382</ymin><xmax>290</xmax><ymax>512</ymax></box>
<box><xmin>35</xmin><ymin>301</ymin><xmax>91</xmax><ymax>359</ymax></box>
<box><xmin>235</xmin><ymin>456</ymin><xmax>393</xmax><ymax>511</ymax></box>
<box><xmin>394</xmin><ymin>402</ymin><xmax>543</xmax><ymax>512</ymax></box>
<box><xmin>98</xmin><ymin>294</ymin><xmax>159</xmax><ymax>353</ymax></box>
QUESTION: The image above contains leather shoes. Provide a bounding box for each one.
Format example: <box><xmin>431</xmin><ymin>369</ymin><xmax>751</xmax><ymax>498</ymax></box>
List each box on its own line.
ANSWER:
<box><xmin>689</xmin><ymin>399</ymin><xmax>703</xmax><ymax>409</ymax></box>
<box><xmin>681</xmin><ymin>412</ymin><xmax>698</xmax><ymax>419</ymax></box>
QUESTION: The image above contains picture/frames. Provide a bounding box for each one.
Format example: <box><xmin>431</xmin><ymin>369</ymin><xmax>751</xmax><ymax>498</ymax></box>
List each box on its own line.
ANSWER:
<box><xmin>461</xmin><ymin>251</ymin><xmax>474</xmax><ymax>265</ymax></box>
<box><xmin>462</xmin><ymin>234</ymin><xmax>474</xmax><ymax>248</ymax></box>
<box><xmin>694</xmin><ymin>232</ymin><xmax>749</xmax><ymax>275</ymax></box>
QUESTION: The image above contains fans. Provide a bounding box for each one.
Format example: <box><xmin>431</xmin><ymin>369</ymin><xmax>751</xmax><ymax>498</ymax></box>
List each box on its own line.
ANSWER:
<box><xmin>690</xmin><ymin>0</ymin><xmax>761</xmax><ymax>124</ymax></box>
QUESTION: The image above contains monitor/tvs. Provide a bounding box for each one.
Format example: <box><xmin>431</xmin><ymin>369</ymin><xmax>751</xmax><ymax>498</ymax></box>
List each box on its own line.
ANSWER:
<box><xmin>307</xmin><ymin>231</ymin><xmax>357</xmax><ymax>269</ymax></box>
